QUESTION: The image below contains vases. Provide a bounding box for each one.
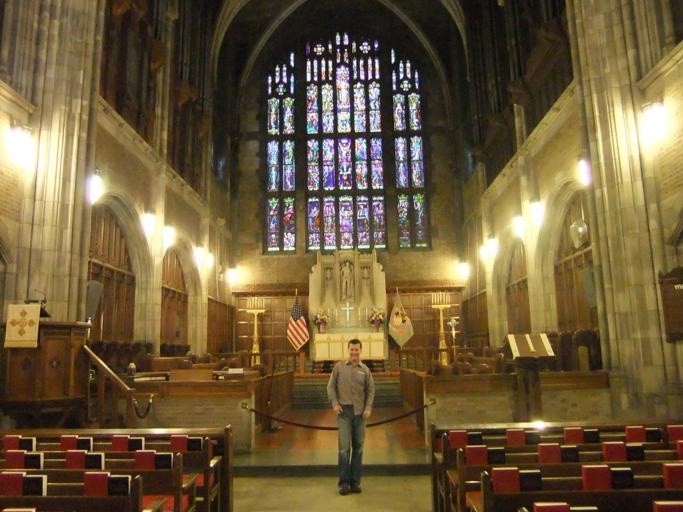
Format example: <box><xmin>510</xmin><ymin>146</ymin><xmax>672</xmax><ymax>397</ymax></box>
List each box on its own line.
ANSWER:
<box><xmin>317</xmin><ymin>322</ymin><xmax>324</xmax><ymax>332</ymax></box>
<box><xmin>372</xmin><ymin>320</ymin><xmax>380</xmax><ymax>332</ymax></box>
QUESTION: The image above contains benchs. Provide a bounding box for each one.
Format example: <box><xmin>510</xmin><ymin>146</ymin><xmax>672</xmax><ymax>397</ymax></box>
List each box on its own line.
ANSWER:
<box><xmin>87</xmin><ymin>337</ymin><xmax>249</xmax><ymax>386</ymax></box>
<box><xmin>422</xmin><ymin>342</ymin><xmax>516</xmax><ymax>377</ymax></box>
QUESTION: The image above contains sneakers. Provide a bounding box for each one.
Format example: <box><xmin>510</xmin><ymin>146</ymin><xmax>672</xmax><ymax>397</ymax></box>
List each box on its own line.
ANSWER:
<box><xmin>339</xmin><ymin>485</ymin><xmax>362</xmax><ymax>494</ymax></box>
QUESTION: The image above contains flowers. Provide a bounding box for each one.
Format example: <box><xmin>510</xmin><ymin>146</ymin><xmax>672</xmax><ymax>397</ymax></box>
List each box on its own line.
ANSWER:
<box><xmin>367</xmin><ymin>306</ymin><xmax>385</xmax><ymax>323</ymax></box>
<box><xmin>311</xmin><ymin>309</ymin><xmax>331</xmax><ymax>323</ymax></box>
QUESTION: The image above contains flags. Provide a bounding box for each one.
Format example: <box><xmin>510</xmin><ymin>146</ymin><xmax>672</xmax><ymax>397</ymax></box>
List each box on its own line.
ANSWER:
<box><xmin>286</xmin><ymin>296</ymin><xmax>311</xmax><ymax>352</ymax></box>
<box><xmin>388</xmin><ymin>295</ymin><xmax>415</xmax><ymax>350</ymax></box>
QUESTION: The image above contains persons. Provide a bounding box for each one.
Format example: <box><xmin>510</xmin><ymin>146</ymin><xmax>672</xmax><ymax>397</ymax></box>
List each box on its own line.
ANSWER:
<box><xmin>326</xmin><ymin>339</ymin><xmax>376</xmax><ymax>495</ymax></box>
<box><xmin>340</xmin><ymin>261</ymin><xmax>352</xmax><ymax>299</ymax></box>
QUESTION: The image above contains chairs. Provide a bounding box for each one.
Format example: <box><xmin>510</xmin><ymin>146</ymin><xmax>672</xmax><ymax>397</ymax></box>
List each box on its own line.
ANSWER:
<box><xmin>423</xmin><ymin>421</ymin><xmax>682</xmax><ymax>512</ymax></box>
<box><xmin>0</xmin><ymin>421</ymin><xmax>234</xmax><ymax>512</ymax></box>
